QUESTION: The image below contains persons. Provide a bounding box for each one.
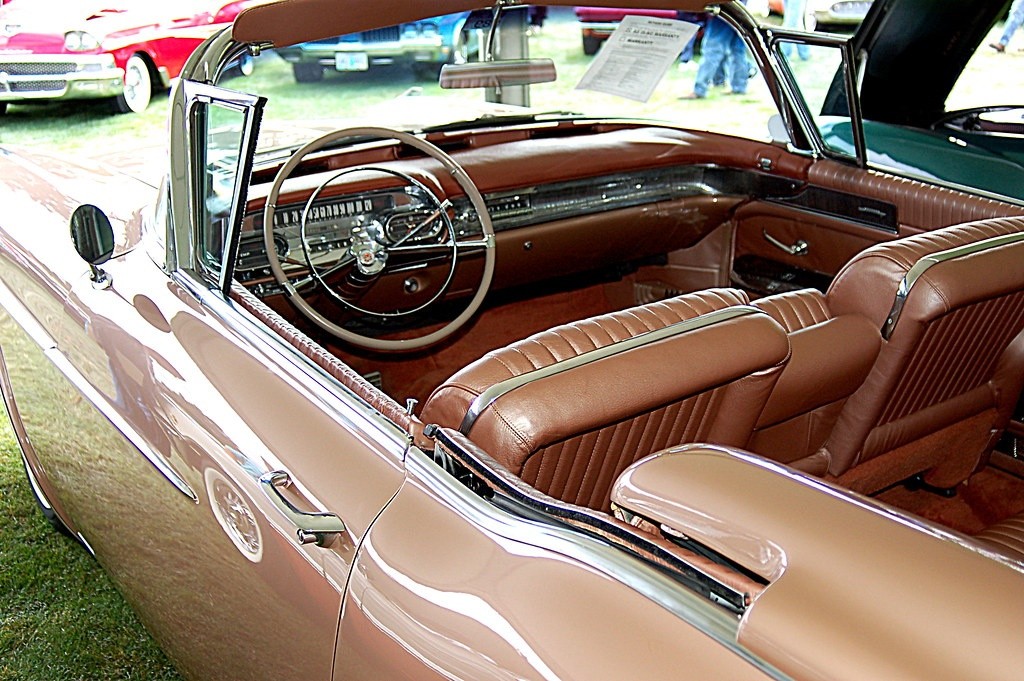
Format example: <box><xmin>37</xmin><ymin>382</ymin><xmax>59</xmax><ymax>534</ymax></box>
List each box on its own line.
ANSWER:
<box><xmin>679</xmin><ymin>0</ymin><xmax>812</xmax><ymax>99</ymax></box>
<box><xmin>989</xmin><ymin>0</ymin><xmax>1024</xmax><ymax>52</ymax></box>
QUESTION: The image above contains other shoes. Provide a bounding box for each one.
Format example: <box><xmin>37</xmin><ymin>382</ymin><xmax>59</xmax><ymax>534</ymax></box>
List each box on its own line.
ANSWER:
<box><xmin>724</xmin><ymin>90</ymin><xmax>745</xmax><ymax>96</ymax></box>
<box><xmin>680</xmin><ymin>93</ymin><xmax>700</xmax><ymax>100</ymax></box>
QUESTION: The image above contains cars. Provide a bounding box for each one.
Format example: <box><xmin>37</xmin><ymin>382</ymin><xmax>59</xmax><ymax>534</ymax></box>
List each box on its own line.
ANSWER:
<box><xmin>0</xmin><ymin>1</ymin><xmax>257</xmax><ymax>114</ymax></box>
<box><xmin>575</xmin><ymin>1</ymin><xmax>746</xmax><ymax>58</ymax></box>
<box><xmin>756</xmin><ymin>1</ymin><xmax>874</xmax><ymax>32</ymax></box>
<box><xmin>272</xmin><ymin>0</ymin><xmax>548</xmax><ymax>83</ymax></box>
<box><xmin>766</xmin><ymin>1</ymin><xmax>1024</xmax><ymax>209</ymax></box>
<box><xmin>0</xmin><ymin>1</ymin><xmax>1024</xmax><ymax>681</ymax></box>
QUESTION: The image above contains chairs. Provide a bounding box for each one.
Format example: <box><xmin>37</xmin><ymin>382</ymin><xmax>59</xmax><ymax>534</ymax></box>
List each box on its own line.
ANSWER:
<box><xmin>416</xmin><ymin>282</ymin><xmax>791</xmax><ymax>509</ymax></box>
<box><xmin>818</xmin><ymin>211</ymin><xmax>1023</xmax><ymax>497</ymax></box>
